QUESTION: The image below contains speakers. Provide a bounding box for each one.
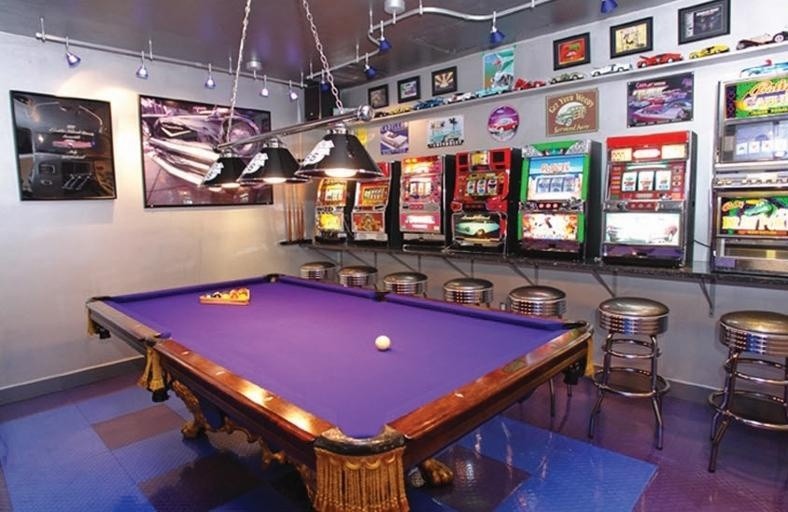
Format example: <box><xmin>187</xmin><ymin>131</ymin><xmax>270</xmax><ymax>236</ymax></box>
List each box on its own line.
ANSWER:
<box><xmin>304</xmin><ymin>82</ymin><xmax>340</xmax><ymax>121</ymax></box>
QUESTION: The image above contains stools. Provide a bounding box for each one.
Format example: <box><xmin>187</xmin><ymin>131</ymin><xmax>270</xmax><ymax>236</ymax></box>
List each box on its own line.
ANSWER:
<box><xmin>708</xmin><ymin>312</ymin><xmax>788</xmax><ymax>473</ymax></box>
<box><xmin>299</xmin><ymin>262</ymin><xmax>337</xmax><ymax>281</ymax></box>
<box><xmin>384</xmin><ymin>272</ymin><xmax>428</xmax><ymax>299</ymax></box>
<box><xmin>587</xmin><ymin>298</ymin><xmax>670</xmax><ymax>447</ymax></box>
<box><xmin>444</xmin><ymin>278</ymin><xmax>493</xmax><ymax>309</ymax></box>
<box><xmin>506</xmin><ymin>286</ymin><xmax>572</xmax><ymax>417</ymax></box>
<box><xmin>336</xmin><ymin>266</ymin><xmax>378</xmax><ymax>290</ymax></box>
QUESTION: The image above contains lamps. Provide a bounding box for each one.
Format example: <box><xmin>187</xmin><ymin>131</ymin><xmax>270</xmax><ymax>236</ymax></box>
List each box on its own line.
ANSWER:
<box><xmin>320</xmin><ymin>69</ymin><xmax>330</xmax><ymax>92</ymax></box>
<box><xmin>261</xmin><ymin>75</ymin><xmax>269</xmax><ymax>97</ymax></box>
<box><xmin>288</xmin><ymin>80</ymin><xmax>299</xmax><ymax>102</ymax></box>
<box><xmin>488</xmin><ymin>11</ymin><xmax>506</xmax><ymax>44</ymax></box>
<box><xmin>378</xmin><ymin>20</ymin><xmax>392</xmax><ymax>53</ymax></box>
<box><xmin>200</xmin><ymin>2</ymin><xmax>387</xmax><ymax>191</ymax></box>
<box><xmin>204</xmin><ymin>63</ymin><xmax>216</xmax><ymax>89</ymax></box>
<box><xmin>136</xmin><ymin>50</ymin><xmax>148</xmax><ymax>80</ymax></box>
<box><xmin>363</xmin><ymin>52</ymin><xmax>377</xmax><ymax>79</ymax></box>
<box><xmin>65</xmin><ymin>37</ymin><xmax>80</xmax><ymax>68</ymax></box>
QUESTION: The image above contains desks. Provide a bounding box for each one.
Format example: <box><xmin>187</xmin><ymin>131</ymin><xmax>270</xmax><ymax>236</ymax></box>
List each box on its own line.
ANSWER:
<box><xmin>85</xmin><ymin>272</ymin><xmax>595</xmax><ymax>512</ymax></box>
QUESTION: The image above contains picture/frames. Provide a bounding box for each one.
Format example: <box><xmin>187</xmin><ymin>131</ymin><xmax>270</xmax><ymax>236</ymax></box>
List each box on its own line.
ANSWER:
<box><xmin>9</xmin><ymin>90</ymin><xmax>117</xmax><ymax>201</ymax></box>
<box><xmin>396</xmin><ymin>75</ymin><xmax>422</xmax><ymax>103</ymax></box>
<box><xmin>431</xmin><ymin>65</ymin><xmax>457</xmax><ymax>95</ymax></box>
<box><xmin>678</xmin><ymin>1</ymin><xmax>730</xmax><ymax>44</ymax></box>
<box><xmin>368</xmin><ymin>83</ymin><xmax>388</xmax><ymax>109</ymax></box>
<box><xmin>609</xmin><ymin>17</ymin><xmax>653</xmax><ymax>58</ymax></box>
<box><xmin>552</xmin><ymin>32</ymin><xmax>591</xmax><ymax>71</ymax></box>
<box><xmin>139</xmin><ymin>94</ymin><xmax>274</xmax><ymax>209</ymax></box>
